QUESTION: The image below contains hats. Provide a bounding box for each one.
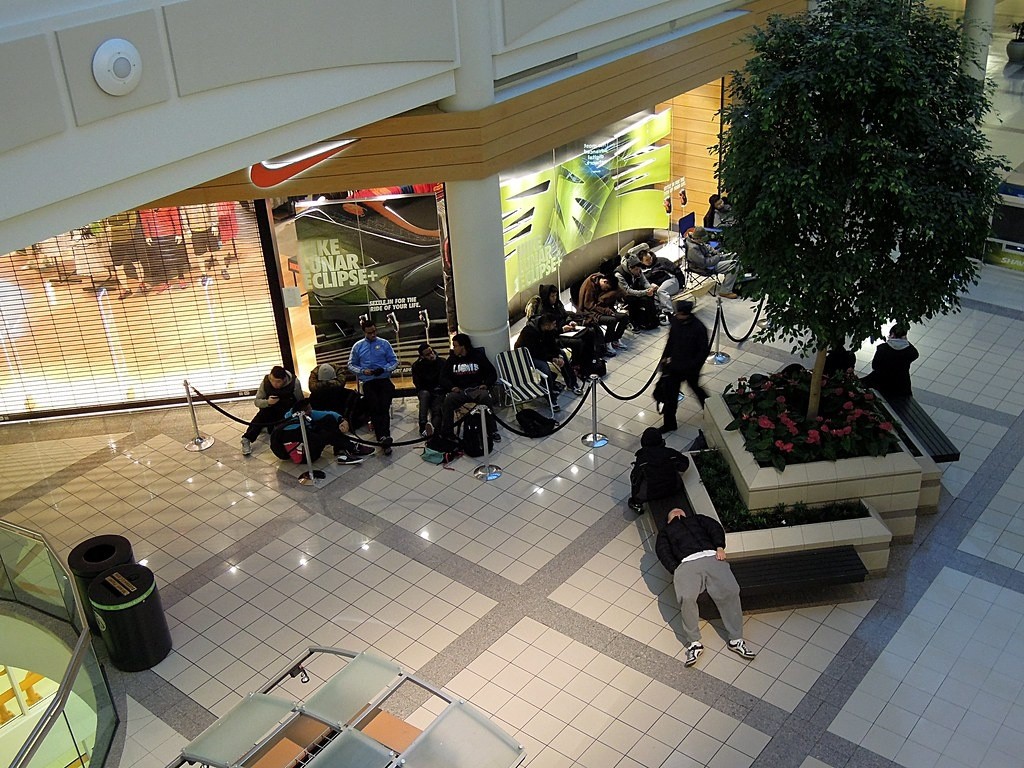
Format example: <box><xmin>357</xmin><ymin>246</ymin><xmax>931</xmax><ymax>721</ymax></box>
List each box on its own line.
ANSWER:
<box><xmin>692</xmin><ymin>225</ymin><xmax>708</xmax><ymax>239</ymax></box>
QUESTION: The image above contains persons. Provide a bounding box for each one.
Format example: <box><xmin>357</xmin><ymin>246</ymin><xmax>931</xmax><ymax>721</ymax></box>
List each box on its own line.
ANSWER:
<box><xmin>281</xmin><ymin>398</ymin><xmax>376</xmax><ymax>465</ymax></box>
<box><xmin>873</xmin><ymin>324</ymin><xmax>919</xmax><ymax>397</ymax></box>
<box><xmin>240</xmin><ymin>366</ymin><xmax>304</xmax><ymax>456</ymax></box>
<box><xmin>308</xmin><ymin>362</ymin><xmax>345</xmax><ymax>417</ymax></box>
<box><xmin>347</xmin><ymin>320</ymin><xmax>400</xmax><ymax>456</ymax></box>
<box><xmin>514</xmin><ymin>193</ymin><xmax>739</xmax><ymax>411</ymax></box>
<box><xmin>657</xmin><ymin>300</ymin><xmax>712</xmax><ymax>433</ymax></box>
<box><xmin>438</xmin><ymin>334</ymin><xmax>501</xmax><ymax>443</ymax></box>
<box><xmin>628</xmin><ymin>427</ymin><xmax>689</xmax><ymax>515</ymax></box>
<box><xmin>412</xmin><ymin>344</ymin><xmax>446</xmax><ymax>437</ymax></box>
<box><xmin>655</xmin><ymin>508</ymin><xmax>756</xmax><ymax>667</ymax></box>
<box><xmin>822</xmin><ymin>333</ymin><xmax>858</xmax><ymax>383</ymax></box>
<box><xmin>99</xmin><ymin>203</ymin><xmax>231</xmax><ymax>302</ymax></box>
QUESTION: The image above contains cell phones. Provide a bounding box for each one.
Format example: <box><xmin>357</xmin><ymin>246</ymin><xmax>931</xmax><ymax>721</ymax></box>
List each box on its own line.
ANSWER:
<box><xmin>270</xmin><ymin>396</ymin><xmax>278</xmax><ymax>399</ymax></box>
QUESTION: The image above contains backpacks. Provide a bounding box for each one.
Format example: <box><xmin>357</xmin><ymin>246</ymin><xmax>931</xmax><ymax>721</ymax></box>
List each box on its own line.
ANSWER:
<box><xmin>271</xmin><ymin>415</ymin><xmax>302</xmax><ymax>461</ymax></box>
<box><xmin>463</xmin><ymin>414</ymin><xmax>493</xmax><ymax>457</ymax></box>
<box><xmin>515</xmin><ymin>405</ymin><xmax>559</xmax><ymax>439</ymax></box>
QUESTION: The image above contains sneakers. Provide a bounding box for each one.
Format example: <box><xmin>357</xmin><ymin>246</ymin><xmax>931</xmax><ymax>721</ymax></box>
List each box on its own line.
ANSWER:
<box><xmin>727</xmin><ymin>640</ymin><xmax>756</xmax><ymax>659</ymax></box>
<box><xmin>241</xmin><ymin>438</ymin><xmax>252</xmax><ymax>455</ymax></box>
<box><xmin>351</xmin><ymin>442</ymin><xmax>376</xmax><ymax>456</ymax></box>
<box><xmin>337</xmin><ymin>450</ymin><xmax>363</xmax><ymax>465</ymax></box>
<box><xmin>684</xmin><ymin>642</ymin><xmax>704</xmax><ymax>668</ymax></box>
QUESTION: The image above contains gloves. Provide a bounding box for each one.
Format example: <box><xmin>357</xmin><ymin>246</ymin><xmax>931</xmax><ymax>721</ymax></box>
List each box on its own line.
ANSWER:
<box><xmin>371</xmin><ymin>368</ymin><xmax>384</xmax><ymax>377</ymax></box>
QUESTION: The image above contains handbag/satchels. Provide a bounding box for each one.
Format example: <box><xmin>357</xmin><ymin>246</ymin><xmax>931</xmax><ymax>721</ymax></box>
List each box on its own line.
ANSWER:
<box><xmin>580</xmin><ymin>357</ymin><xmax>606</xmax><ymax>377</ymax></box>
<box><xmin>413</xmin><ymin>434</ymin><xmax>463</xmax><ymax>471</ymax></box>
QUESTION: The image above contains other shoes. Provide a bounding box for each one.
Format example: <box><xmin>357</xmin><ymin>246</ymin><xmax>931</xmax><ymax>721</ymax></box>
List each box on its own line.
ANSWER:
<box><xmin>490</xmin><ymin>432</ymin><xmax>501</xmax><ymax>442</ymax></box>
<box><xmin>118</xmin><ymin>288</ymin><xmax>133</xmax><ymax>300</ymax></box>
<box><xmin>719</xmin><ymin>293</ymin><xmax>738</xmax><ymax>299</ymax></box>
<box><xmin>200</xmin><ymin>274</ymin><xmax>208</xmax><ymax>286</ymax></box>
<box><xmin>628</xmin><ymin>497</ymin><xmax>644</xmax><ymax>515</ymax></box>
<box><xmin>547</xmin><ymin>402</ymin><xmax>561</xmax><ymax>412</ymax></box>
<box><xmin>139</xmin><ymin>284</ymin><xmax>149</xmax><ymax>295</ymax></box>
<box><xmin>659</xmin><ymin>311</ymin><xmax>674</xmax><ymax>325</ymax></box>
<box><xmin>613</xmin><ymin>343</ymin><xmax>627</xmax><ymax>347</ymax></box>
<box><xmin>29</xmin><ymin>260</ymin><xmax>38</xmax><ymax>272</ymax></box>
<box><xmin>221</xmin><ymin>268</ymin><xmax>230</xmax><ymax>280</ymax></box>
<box><xmin>625</xmin><ymin>322</ymin><xmax>640</xmax><ymax>334</ymax></box>
<box><xmin>42</xmin><ymin>259</ymin><xmax>52</xmax><ymax>268</ymax></box>
<box><xmin>572</xmin><ymin>385</ymin><xmax>583</xmax><ymax>395</ymax></box>
<box><xmin>378</xmin><ymin>436</ymin><xmax>393</xmax><ymax>457</ymax></box>
<box><xmin>158</xmin><ymin>281</ymin><xmax>171</xmax><ymax>293</ymax></box>
<box><xmin>422</xmin><ymin>421</ymin><xmax>434</xmax><ymax>438</ymax></box>
<box><xmin>178</xmin><ymin>279</ymin><xmax>189</xmax><ymax>290</ymax></box>
<box><xmin>657</xmin><ymin>424</ymin><xmax>678</xmax><ymax>433</ymax></box>
<box><xmin>606</xmin><ymin>347</ymin><xmax>616</xmax><ymax>353</ymax></box>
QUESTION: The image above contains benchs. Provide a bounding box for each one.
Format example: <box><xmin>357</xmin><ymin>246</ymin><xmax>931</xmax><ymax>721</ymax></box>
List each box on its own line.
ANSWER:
<box><xmin>884</xmin><ymin>394</ymin><xmax>962</xmax><ymax>463</ymax></box>
<box><xmin>647</xmin><ymin>478</ymin><xmax>869</xmax><ymax>619</ymax></box>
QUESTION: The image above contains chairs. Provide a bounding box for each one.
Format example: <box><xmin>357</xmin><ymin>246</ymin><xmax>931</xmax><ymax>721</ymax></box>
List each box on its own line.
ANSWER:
<box><xmin>627</xmin><ymin>242</ymin><xmax>698</xmax><ymax>316</ymax></box>
<box><xmin>496</xmin><ymin>346</ymin><xmax>556</xmax><ymax>424</ymax></box>
<box><xmin>678</xmin><ymin>211</ymin><xmax>723</xmax><ymax>297</ymax></box>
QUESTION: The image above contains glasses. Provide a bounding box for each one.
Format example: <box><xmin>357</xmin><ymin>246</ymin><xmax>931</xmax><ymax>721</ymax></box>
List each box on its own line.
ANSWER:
<box><xmin>364</xmin><ymin>329</ymin><xmax>376</xmax><ymax>334</ymax></box>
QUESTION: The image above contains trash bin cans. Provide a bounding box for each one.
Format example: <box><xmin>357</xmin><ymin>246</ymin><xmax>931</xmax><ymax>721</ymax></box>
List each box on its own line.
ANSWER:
<box><xmin>67</xmin><ymin>534</ymin><xmax>136</xmax><ymax>620</ymax></box>
<box><xmin>88</xmin><ymin>563</ymin><xmax>173</xmax><ymax>674</ymax></box>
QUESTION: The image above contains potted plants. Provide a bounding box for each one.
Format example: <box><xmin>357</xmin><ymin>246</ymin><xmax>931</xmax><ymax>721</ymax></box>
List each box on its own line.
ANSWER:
<box><xmin>1006</xmin><ymin>20</ymin><xmax>1024</xmax><ymax>63</ymax></box>
